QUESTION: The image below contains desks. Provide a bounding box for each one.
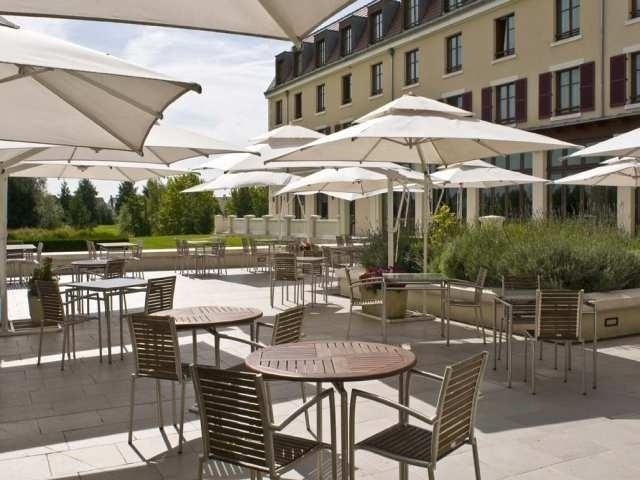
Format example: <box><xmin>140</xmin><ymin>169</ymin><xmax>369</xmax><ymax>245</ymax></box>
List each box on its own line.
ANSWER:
<box><xmin>493</xmin><ymin>295</ymin><xmax>598</xmax><ymax>390</ymax></box>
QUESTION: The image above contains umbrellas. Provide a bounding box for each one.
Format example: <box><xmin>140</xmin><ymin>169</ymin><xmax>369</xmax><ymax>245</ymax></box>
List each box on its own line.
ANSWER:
<box><xmin>1</xmin><ymin>1</ymin><xmax>356</xmax><ymax>54</ymax></box>
<box><xmin>545</xmin><ymin>155</ymin><xmax>640</xmax><ymax>188</ymax></box>
<box><xmin>7</xmin><ymin>159</ymin><xmax>193</xmax><ymax>183</ymax></box>
<box><xmin>1</xmin><ymin>121</ymin><xmax>262</xmax><ymax>331</ymax></box>
<box><xmin>559</xmin><ymin>124</ymin><xmax>640</xmax><ymax>160</ymax></box>
<box><xmin>0</xmin><ymin>13</ymin><xmax>203</xmax><ymax>158</ymax></box>
